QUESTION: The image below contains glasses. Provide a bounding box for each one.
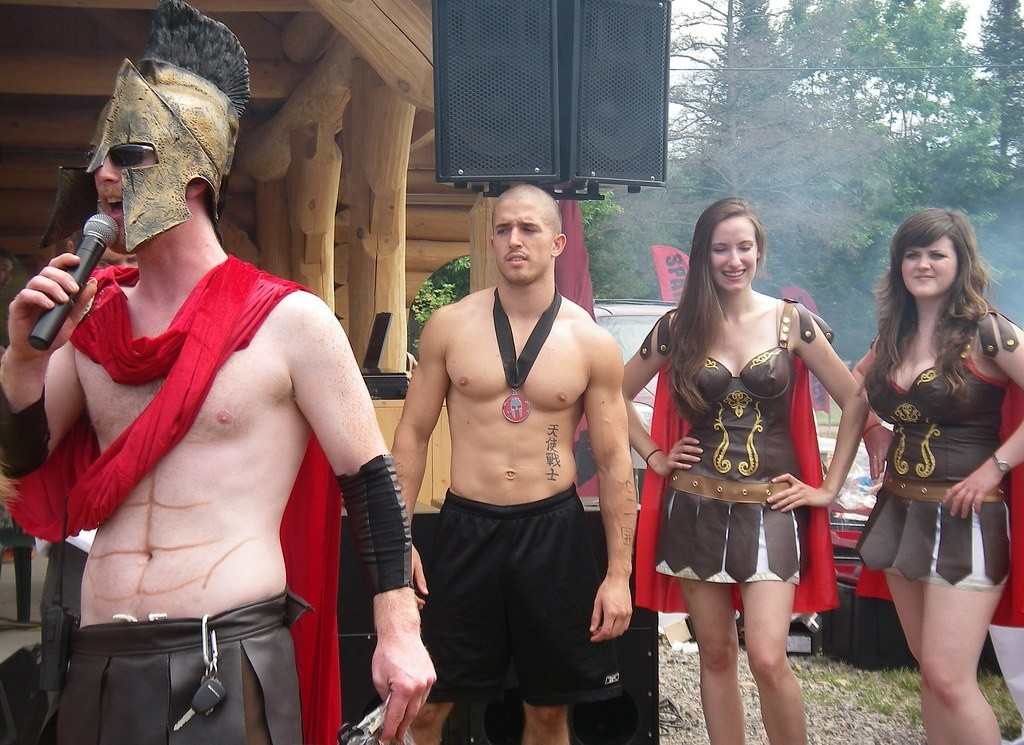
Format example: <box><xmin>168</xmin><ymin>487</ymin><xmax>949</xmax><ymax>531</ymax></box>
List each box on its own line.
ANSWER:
<box><xmin>87</xmin><ymin>144</ymin><xmax>154</xmax><ymax>168</ymax></box>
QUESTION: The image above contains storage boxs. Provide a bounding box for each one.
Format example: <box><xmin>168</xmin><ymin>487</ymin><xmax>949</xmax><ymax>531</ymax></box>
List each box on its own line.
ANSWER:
<box><xmin>787</xmin><ymin>611</ymin><xmax>821</xmax><ymax>655</ymax></box>
<box><xmin>821</xmin><ymin>580</ymin><xmax>919</xmax><ymax>672</ymax></box>
<box><xmin>664</xmin><ymin>609</ymin><xmax>744</xmax><ymax>645</ymax></box>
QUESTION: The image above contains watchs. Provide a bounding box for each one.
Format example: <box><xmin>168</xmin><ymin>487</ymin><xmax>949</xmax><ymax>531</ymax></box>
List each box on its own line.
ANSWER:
<box><xmin>992</xmin><ymin>453</ymin><xmax>1010</xmax><ymax>472</ymax></box>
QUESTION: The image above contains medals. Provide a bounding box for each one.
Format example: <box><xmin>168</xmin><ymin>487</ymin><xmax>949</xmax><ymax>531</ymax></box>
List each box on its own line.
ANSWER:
<box><xmin>502</xmin><ymin>393</ymin><xmax>531</xmax><ymax>422</ymax></box>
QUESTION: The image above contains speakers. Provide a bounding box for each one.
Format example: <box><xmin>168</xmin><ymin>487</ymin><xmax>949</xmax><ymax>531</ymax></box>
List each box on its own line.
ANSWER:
<box><xmin>431</xmin><ymin>0</ymin><xmax>671</xmax><ymax>193</ymax></box>
<box><xmin>339</xmin><ymin>496</ymin><xmax>659</xmax><ymax>745</ymax></box>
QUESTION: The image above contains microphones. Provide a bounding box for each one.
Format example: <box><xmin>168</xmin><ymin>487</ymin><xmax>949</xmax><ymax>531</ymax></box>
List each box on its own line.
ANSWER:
<box><xmin>26</xmin><ymin>214</ymin><xmax>119</xmax><ymax>351</ymax></box>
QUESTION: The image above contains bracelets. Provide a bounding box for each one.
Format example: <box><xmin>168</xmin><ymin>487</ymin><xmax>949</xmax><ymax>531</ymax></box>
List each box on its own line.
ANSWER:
<box><xmin>646</xmin><ymin>448</ymin><xmax>662</xmax><ymax>465</ymax></box>
<box><xmin>863</xmin><ymin>423</ymin><xmax>881</xmax><ymax>439</ymax></box>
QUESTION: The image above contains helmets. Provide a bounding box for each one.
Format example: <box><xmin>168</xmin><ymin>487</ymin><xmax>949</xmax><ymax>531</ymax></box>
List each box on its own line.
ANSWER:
<box><xmin>39</xmin><ymin>0</ymin><xmax>249</xmax><ymax>252</ymax></box>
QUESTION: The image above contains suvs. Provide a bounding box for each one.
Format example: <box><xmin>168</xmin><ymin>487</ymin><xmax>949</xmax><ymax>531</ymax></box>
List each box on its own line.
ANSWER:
<box><xmin>587</xmin><ymin>296</ymin><xmax>688</xmax><ymax>442</ymax></box>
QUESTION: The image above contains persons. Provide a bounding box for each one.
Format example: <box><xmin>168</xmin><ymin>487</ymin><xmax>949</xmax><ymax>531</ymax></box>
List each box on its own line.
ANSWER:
<box><xmin>0</xmin><ymin>1</ymin><xmax>438</xmax><ymax>745</ymax></box>
<box><xmin>388</xmin><ymin>183</ymin><xmax>637</xmax><ymax>745</ymax></box>
<box><xmin>618</xmin><ymin>196</ymin><xmax>869</xmax><ymax>744</ymax></box>
<box><xmin>848</xmin><ymin>207</ymin><xmax>1024</xmax><ymax>745</ymax></box>
